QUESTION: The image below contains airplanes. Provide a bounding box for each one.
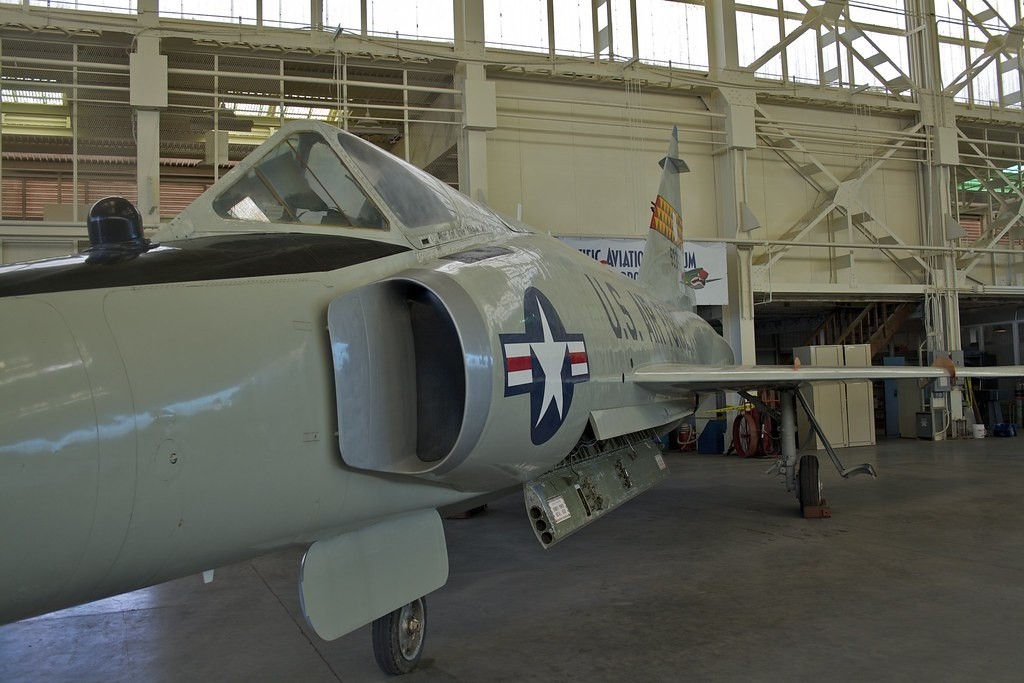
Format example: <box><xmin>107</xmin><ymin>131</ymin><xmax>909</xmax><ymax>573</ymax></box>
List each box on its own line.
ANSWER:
<box><xmin>0</xmin><ymin>118</ymin><xmax>1024</xmax><ymax>683</ymax></box>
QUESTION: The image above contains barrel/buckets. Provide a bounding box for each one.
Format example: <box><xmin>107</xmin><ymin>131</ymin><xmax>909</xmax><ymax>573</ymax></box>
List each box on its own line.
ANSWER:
<box><xmin>972</xmin><ymin>423</ymin><xmax>984</xmax><ymax>438</ymax></box>
